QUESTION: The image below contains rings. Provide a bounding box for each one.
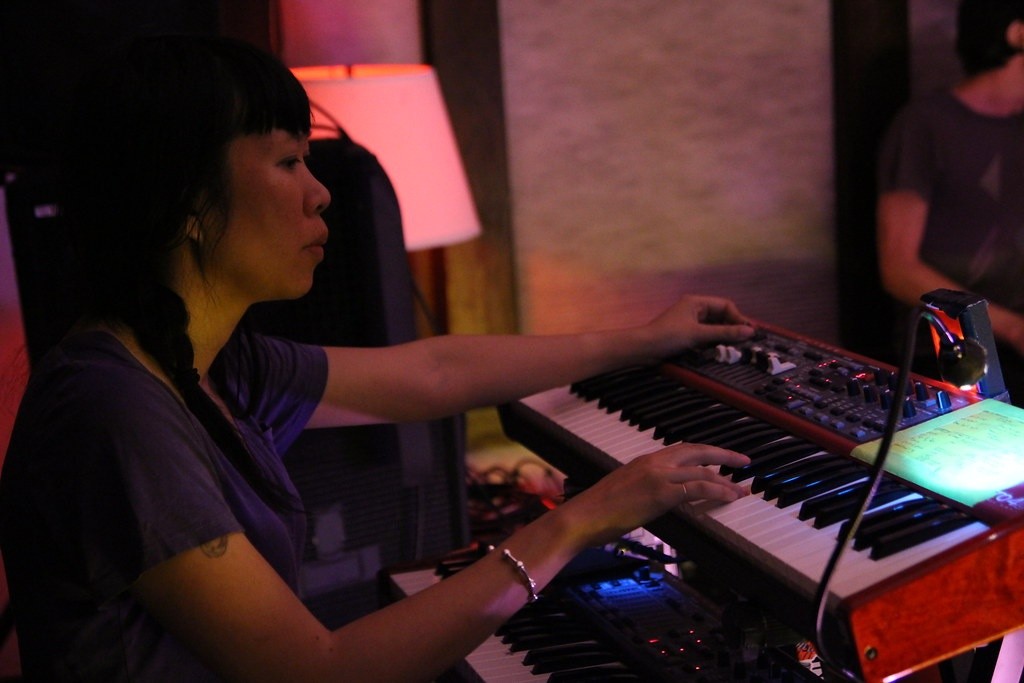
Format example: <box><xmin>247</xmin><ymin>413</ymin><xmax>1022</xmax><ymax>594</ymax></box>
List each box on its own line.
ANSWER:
<box><xmin>681</xmin><ymin>482</ymin><xmax>688</xmax><ymax>497</ymax></box>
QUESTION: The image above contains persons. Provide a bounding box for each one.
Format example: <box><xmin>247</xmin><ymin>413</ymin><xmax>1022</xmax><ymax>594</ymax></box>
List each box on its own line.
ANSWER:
<box><xmin>875</xmin><ymin>12</ymin><xmax>1024</xmax><ymax>345</ymax></box>
<box><xmin>0</xmin><ymin>18</ymin><xmax>761</xmax><ymax>683</ymax></box>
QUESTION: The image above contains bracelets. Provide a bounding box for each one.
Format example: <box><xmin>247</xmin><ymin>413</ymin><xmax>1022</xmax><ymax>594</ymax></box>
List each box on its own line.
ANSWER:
<box><xmin>484</xmin><ymin>544</ymin><xmax>537</xmax><ymax>605</ymax></box>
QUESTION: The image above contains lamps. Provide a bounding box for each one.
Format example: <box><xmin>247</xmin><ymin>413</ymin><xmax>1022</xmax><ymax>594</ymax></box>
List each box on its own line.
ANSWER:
<box><xmin>285</xmin><ymin>63</ymin><xmax>484</xmax><ymax>252</ymax></box>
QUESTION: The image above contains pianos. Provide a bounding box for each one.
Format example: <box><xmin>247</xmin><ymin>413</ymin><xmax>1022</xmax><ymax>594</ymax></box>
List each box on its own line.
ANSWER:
<box><xmin>391</xmin><ymin>308</ymin><xmax>1024</xmax><ymax>683</ymax></box>
